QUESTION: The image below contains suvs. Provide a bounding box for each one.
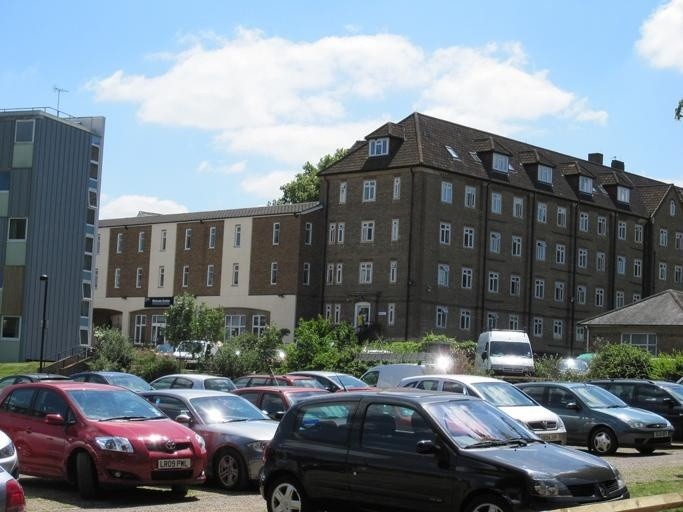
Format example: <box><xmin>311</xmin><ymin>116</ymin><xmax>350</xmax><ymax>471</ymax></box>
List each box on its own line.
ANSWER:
<box><xmin>257</xmin><ymin>389</ymin><xmax>630</xmax><ymax>511</ymax></box>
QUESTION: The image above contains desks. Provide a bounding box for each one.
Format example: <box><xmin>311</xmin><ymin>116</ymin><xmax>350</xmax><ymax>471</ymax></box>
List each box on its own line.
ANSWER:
<box><xmin>40</xmin><ymin>273</ymin><xmax>49</xmax><ymax>373</ymax></box>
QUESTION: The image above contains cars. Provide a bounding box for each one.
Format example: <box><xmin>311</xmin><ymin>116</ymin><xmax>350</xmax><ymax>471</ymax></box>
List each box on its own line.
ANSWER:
<box><xmin>70</xmin><ymin>371</ymin><xmax>157</xmax><ymax>394</ymax></box>
<box><xmin>234</xmin><ymin>374</ymin><xmax>327</xmax><ymax>390</ymax></box>
<box><xmin>395</xmin><ymin>374</ymin><xmax>568</xmax><ymax>446</ymax></box>
<box><xmin>554</xmin><ymin>358</ymin><xmax>590</xmax><ymax>375</ymax></box>
<box><xmin>1</xmin><ymin>467</ymin><xmax>27</xmax><ymax>510</ymax></box>
<box><xmin>512</xmin><ymin>381</ymin><xmax>675</xmax><ymax>457</ymax></box>
<box><xmin>149</xmin><ymin>373</ymin><xmax>238</xmax><ymax>392</ymax></box>
<box><xmin>2</xmin><ymin>373</ymin><xmax>75</xmax><ymax>389</ymax></box>
<box><xmin>585</xmin><ymin>379</ymin><xmax>681</xmax><ymax>443</ymax></box>
<box><xmin>285</xmin><ymin>371</ymin><xmax>371</xmax><ymax>392</ymax></box>
<box><xmin>137</xmin><ymin>389</ymin><xmax>281</xmax><ymax>491</ymax></box>
<box><xmin>2</xmin><ymin>430</ymin><xmax>21</xmax><ymax>481</ymax></box>
<box><xmin>231</xmin><ymin>386</ymin><xmax>351</xmax><ymax>424</ymax></box>
<box><xmin>152</xmin><ymin>339</ymin><xmax>218</xmax><ymax>369</ymax></box>
<box><xmin>2</xmin><ymin>382</ymin><xmax>209</xmax><ymax>501</ymax></box>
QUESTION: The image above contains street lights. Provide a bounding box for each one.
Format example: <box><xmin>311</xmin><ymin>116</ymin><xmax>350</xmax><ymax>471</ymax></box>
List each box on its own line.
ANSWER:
<box><xmin>40</xmin><ymin>273</ymin><xmax>49</xmax><ymax>373</ymax></box>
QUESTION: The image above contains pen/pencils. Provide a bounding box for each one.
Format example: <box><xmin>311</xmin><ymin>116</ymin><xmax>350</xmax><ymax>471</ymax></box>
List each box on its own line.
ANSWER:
<box><xmin>476</xmin><ymin>330</ymin><xmax>535</xmax><ymax>375</ymax></box>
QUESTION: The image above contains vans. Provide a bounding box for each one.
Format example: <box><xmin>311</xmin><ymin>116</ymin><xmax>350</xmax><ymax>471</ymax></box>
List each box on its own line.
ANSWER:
<box><xmin>359</xmin><ymin>365</ymin><xmax>449</xmax><ymax>389</ymax></box>
<box><xmin>476</xmin><ymin>330</ymin><xmax>535</xmax><ymax>375</ymax></box>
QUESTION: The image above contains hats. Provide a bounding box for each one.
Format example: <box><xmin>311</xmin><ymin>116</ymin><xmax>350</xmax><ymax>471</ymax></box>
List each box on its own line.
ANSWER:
<box><xmin>554</xmin><ymin>358</ymin><xmax>590</xmax><ymax>375</ymax></box>
<box><xmin>152</xmin><ymin>339</ymin><xmax>218</xmax><ymax>369</ymax></box>
